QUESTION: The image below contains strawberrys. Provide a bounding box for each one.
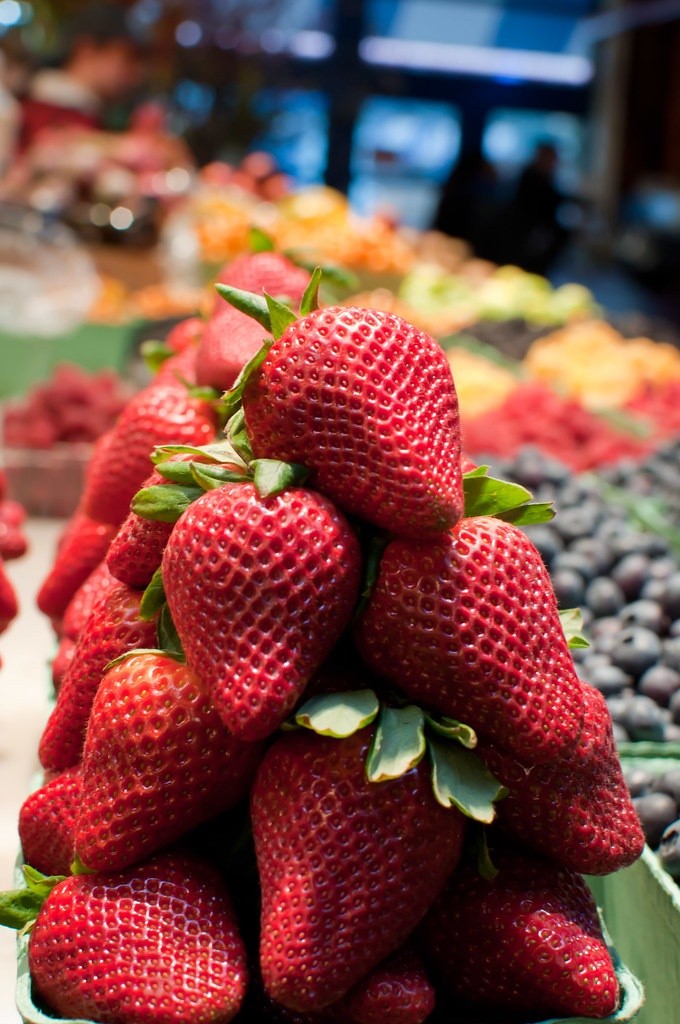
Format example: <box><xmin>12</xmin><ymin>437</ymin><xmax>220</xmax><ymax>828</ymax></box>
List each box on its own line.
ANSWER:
<box><xmin>0</xmin><ymin>216</ymin><xmax>644</xmax><ymax>1024</ymax></box>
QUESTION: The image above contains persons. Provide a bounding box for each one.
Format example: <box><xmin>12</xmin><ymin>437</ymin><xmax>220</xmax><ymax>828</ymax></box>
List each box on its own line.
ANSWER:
<box><xmin>503</xmin><ymin>140</ymin><xmax>565</xmax><ymax>278</ymax></box>
<box><xmin>430</xmin><ymin>144</ymin><xmax>511</xmax><ymax>267</ymax></box>
